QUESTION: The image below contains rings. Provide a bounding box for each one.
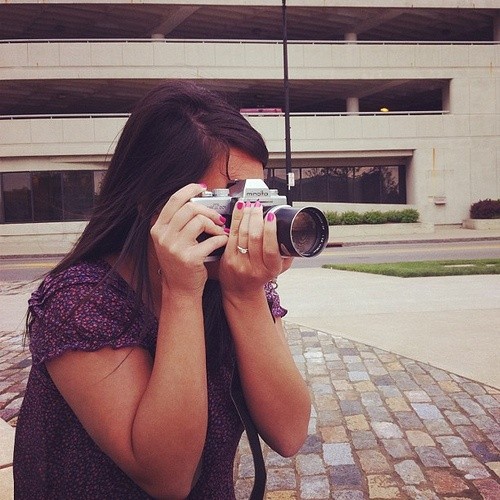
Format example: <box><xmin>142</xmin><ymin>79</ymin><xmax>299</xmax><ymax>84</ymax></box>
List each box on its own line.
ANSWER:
<box><xmin>236</xmin><ymin>245</ymin><xmax>250</xmax><ymax>254</ymax></box>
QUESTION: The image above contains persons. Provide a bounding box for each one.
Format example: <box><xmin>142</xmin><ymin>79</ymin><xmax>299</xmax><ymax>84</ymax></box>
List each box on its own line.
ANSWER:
<box><xmin>11</xmin><ymin>78</ymin><xmax>313</xmax><ymax>500</ymax></box>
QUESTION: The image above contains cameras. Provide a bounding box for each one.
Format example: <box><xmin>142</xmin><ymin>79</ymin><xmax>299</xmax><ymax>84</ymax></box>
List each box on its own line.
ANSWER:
<box><xmin>190</xmin><ymin>178</ymin><xmax>328</xmax><ymax>263</ymax></box>
